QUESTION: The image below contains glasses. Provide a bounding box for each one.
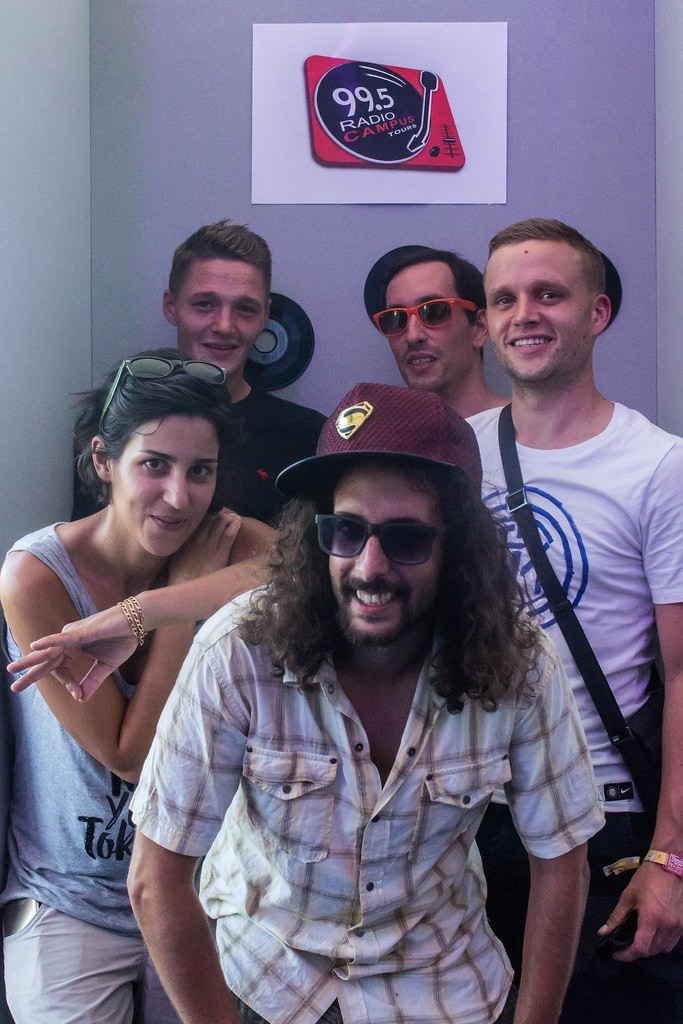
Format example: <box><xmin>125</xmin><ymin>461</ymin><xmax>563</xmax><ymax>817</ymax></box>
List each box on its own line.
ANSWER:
<box><xmin>98</xmin><ymin>357</ymin><xmax>228</xmax><ymax>433</ymax></box>
<box><xmin>372</xmin><ymin>298</ymin><xmax>478</xmax><ymax>337</ymax></box>
<box><xmin>315</xmin><ymin>514</ymin><xmax>448</xmax><ymax>565</ymax></box>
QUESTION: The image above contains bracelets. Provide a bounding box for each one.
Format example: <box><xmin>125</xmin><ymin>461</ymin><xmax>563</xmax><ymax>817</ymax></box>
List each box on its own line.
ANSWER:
<box><xmin>117</xmin><ymin>596</ymin><xmax>147</xmax><ymax>646</ymax></box>
<box><xmin>644</xmin><ymin>850</ymin><xmax>683</xmax><ymax>877</ymax></box>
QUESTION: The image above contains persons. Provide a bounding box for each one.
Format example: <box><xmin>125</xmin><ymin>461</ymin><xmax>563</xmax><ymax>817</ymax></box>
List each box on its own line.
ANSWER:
<box><xmin>0</xmin><ymin>357</ymin><xmax>281</xmax><ymax>1024</ymax></box>
<box><xmin>127</xmin><ymin>382</ymin><xmax>606</xmax><ymax>1024</ymax></box>
<box><xmin>70</xmin><ymin>216</ymin><xmax>328</xmax><ymax>532</ymax></box>
<box><xmin>364</xmin><ymin>246</ymin><xmax>512</xmax><ymax>419</ymax></box>
<box><xmin>465</xmin><ymin>218</ymin><xmax>683</xmax><ymax>1024</ymax></box>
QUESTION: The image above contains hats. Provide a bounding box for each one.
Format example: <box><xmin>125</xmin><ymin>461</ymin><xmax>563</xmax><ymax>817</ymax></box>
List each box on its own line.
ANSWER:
<box><xmin>274</xmin><ymin>381</ymin><xmax>483</xmax><ymax>501</ymax></box>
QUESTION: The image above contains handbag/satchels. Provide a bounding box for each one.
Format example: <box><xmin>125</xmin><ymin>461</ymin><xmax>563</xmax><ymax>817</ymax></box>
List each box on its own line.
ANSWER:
<box><xmin>613</xmin><ymin>686</ymin><xmax>665</xmax><ymax>823</ymax></box>
